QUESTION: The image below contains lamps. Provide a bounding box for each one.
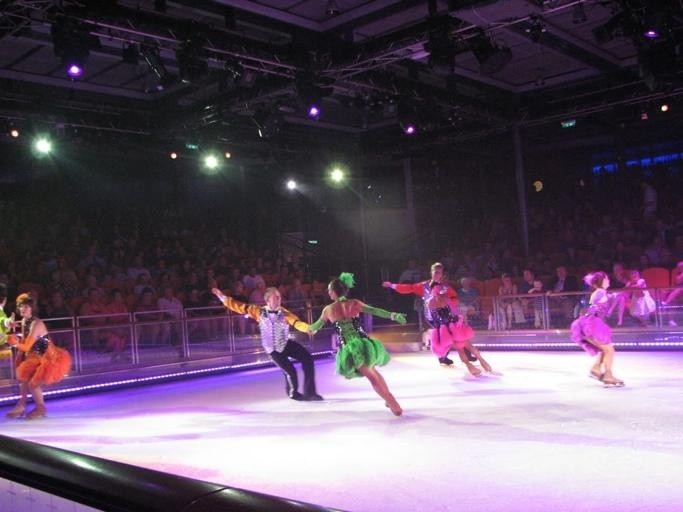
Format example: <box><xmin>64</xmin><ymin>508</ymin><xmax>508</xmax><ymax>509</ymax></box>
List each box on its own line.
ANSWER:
<box><xmin>47</xmin><ymin>1</ymin><xmax>683</xmax><ymax>162</ymax></box>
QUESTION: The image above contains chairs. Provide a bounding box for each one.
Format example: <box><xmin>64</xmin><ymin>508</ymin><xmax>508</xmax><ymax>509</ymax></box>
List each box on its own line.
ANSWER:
<box><xmin>0</xmin><ymin>251</ymin><xmax>339</xmax><ymax>353</ymax></box>
<box><xmin>445</xmin><ymin>217</ymin><xmax>683</xmax><ymax>324</ymax></box>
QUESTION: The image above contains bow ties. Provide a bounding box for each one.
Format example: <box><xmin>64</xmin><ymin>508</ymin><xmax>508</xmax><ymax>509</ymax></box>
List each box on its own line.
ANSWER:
<box><xmin>268</xmin><ymin>310</ymin><xmax>278</xmax><ymax>313</ymax></box>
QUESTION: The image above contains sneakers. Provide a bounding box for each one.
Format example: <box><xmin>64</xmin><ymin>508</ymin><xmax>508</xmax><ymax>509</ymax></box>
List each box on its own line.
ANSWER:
<box><xmin>292</xmin><ymin>391</ymin><xmax>303</xmax><ymax>400</ymax></box>
<box><xmin>440</xmin><ymin>358</ymin><xmax>491</xmax><ymax>374</ymax></box>
<box><xmin>385</xmin><ymin>394</ymin><xmax>401</xmax><ymax>415</ymax></box>
<box><xmin>27</xmin><ymin>405</ymin><xmax>46</xmax><ymax>415</ymax></box>
<box><xmin>304</xmin><ymin>395</ymin><xmax>321</xmax><ymax>400</ymax></box>
<box><xmin>592</xmin><ymin>366</ymin><xmax>622</xmax><ymax>382</ymax></box>
<box><xmin>8</xmin><ymin>405</ymin><xmax>24</xmax><ymax>415</ymax></box>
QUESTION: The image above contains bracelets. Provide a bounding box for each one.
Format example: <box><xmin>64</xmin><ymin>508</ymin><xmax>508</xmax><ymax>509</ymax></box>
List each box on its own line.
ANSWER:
<box><xmin>12</xmin><ymin>342</ymin><xmax>18</xmax><ymax>348</ymax></box>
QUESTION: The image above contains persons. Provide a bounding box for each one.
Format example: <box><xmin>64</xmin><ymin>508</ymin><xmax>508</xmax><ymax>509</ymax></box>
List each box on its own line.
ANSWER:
<box><xmin>570</xmin><ymin>271</ymin><xmax>630</xmax><ymax>386</ymax></box>
<box><xmin>284</xmin><ymin>272</ymin><xmax>410</xmax><ymax>419</ymax></box>
<box><xmin>461</xmin><ymin>179</ymin><xmax>681</xmax><ymax>327</ymax></box>
<box><xmin>0</xmin><ymin>196</ymin><xmax>340</xmax><ymax>366</ymax></box>
<box><xmin>422</xmin><ymin>280</ymin><xmax>491</xmax><ymax>379</ymax></box>
<box><xmin>382</xmin><ymin>260</ymin><xmax>478</xmax><ymax>365</ymax></box>
<box><xmin>0</xmin><ymin>283</ymin><xmax>15</xmax><ymax>345</ymax></box>
<box><xmin>6</xmin><ymin>293</ymin><xmax>73</xmax><ymax>418</ymax></box>
<box><xmin>213</xmin><ymin>284</ymin><xmax>319</xmax><ymax>400</ymax></box>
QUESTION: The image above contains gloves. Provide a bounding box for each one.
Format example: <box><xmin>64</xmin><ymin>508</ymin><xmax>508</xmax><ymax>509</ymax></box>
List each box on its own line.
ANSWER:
<box><xmin>8</xmin><ymin>335</ymin><xmax>36</xmax><ymax>352</ymax></box>
<box><xmin>363</xmin><ymin>304</ymin><xmax>407</xmax><ymax>324</ymax></box>
